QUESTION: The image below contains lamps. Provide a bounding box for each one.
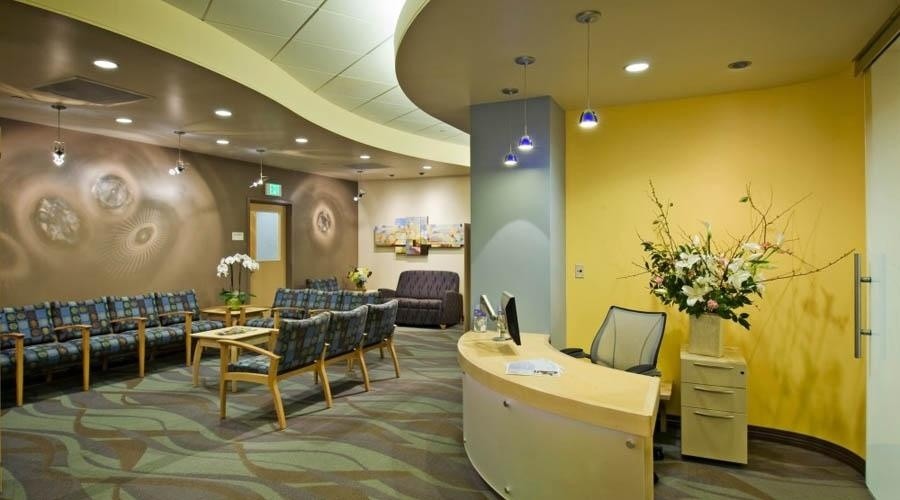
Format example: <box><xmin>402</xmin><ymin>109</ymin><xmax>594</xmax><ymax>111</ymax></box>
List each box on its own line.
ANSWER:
<box><xmin>515</xmin><ymin>56</ymin><xmax>536</xmax><ymax>151</ymax></box>
<box><xmin>576</xmin><ymin>11</ymin><xmax>601</xmax><ymax>128</ymax></box>
<box><xmin>257</xmin><ymin>150</ymin><xmax>266</xmax><ymax>185</ymax></box>
<box><xmin>174</xmin><ymin>131</ymin><xmax>185</xmax><ymax>171</ymax></box>
<box><xmin>51</xmin><ymin>104</ymin><xmax>66</xmax><ymax>173</ymax></box>
<box><xmin>358</xmin><ymin>171</ymin><xmax>363</xmax><ymax>199</ymax></box>
<box><xmin>502</xmin><ymin>88</ymin><xmax>519</xmax><ymax>167</ymax></box>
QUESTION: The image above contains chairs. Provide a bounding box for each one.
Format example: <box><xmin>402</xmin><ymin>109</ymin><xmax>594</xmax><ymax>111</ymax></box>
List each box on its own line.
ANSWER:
<box><xmin>241</xmin><ymin>278</ymin><xmax>377</xmax><ymax>329</ymax></box>
<box><xmin>559</xmin><ymin>304</ymin><xmax>666</xmax><ymax>486</ymax></box>
<box><xmin>322</xmin><ymin>304</ymin><xmax>371</xmax><ymax>408</ymax></box>
<box><xmin>364</xmin><ymin>299</ymin><xmax>400</xmax><ymax>387</ymax></box>
<box><xmin>0</xmin><ymin>288</ymin><xmax>225</xmax><ymax>407</ymax></box>
<box><xmin>216</xmin><ymin>310</ymin><xmax>333</xmax><ymax>430</ymax></box>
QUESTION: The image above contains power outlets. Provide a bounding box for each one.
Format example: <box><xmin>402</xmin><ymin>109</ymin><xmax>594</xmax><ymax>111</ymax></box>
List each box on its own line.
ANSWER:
<box><xmin>576</xmin><ymin>265</ymin><xmax>585</xmax><ymax>278</ymax></box>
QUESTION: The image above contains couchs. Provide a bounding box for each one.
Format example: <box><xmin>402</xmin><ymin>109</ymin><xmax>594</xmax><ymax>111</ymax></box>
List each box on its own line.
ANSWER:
<box><xmin>379</xmin><ymin>269</ymin><xmax>463</xmax><ymax>329</ymax></box>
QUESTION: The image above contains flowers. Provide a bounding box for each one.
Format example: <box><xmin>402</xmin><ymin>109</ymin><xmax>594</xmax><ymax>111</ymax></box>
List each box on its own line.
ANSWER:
<box><xmin>615</xmin><ymin>173</ymin><xmax>855</xmax><ymax>330</ymax></box>
<box><xmin>347</xmin><ymin>267</ymin><xmax>372</xmax><ymax>286</ymax></box>
<box><xmin>216</xmin><ymin>253</ymin><xmax>259</xmax><ymax>304</ymax></box>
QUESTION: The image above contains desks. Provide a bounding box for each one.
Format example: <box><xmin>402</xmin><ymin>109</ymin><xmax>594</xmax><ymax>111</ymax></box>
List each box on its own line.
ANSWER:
<box><xmin>199</xmin><ymin>305</ymin><xmax>270</xmax><ymax>323</ymax></box>
<box><xmin>458</xmin><ymin>331</ymin><xmax>659</xmax><ymax>499</ymax></box>
<box><xmin>187</xmin><ymin>325</ymin><xmax>274</xmax><ymax>386</ymax></box>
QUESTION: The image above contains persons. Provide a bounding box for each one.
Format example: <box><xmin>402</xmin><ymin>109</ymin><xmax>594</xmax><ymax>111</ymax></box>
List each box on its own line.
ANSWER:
<box><xmin>474</xmin><ymin>316</ymin><xmax>485</xmax><ymax>331</ymax></box>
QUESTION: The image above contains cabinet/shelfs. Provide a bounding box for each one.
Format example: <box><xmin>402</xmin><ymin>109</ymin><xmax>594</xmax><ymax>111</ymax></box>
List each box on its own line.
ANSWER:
<box><xmin>680</xmin><ymin>344</ymin><xmax>747</xmax><ymax>466</ymax></box>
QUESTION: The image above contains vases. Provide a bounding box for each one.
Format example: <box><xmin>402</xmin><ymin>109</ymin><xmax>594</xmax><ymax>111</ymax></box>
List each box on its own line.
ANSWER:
<box><xmin>688</xmin><ymin>315</ymin><xmax>725</xmax><ymax>357</ymax></box>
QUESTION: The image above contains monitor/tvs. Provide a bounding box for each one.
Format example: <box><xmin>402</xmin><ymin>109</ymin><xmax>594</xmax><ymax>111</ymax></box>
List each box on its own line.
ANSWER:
<box><xmin>501</xmin><ymin>291</ymin><xmax>521</xmax><ymax>346</ymax></box>
